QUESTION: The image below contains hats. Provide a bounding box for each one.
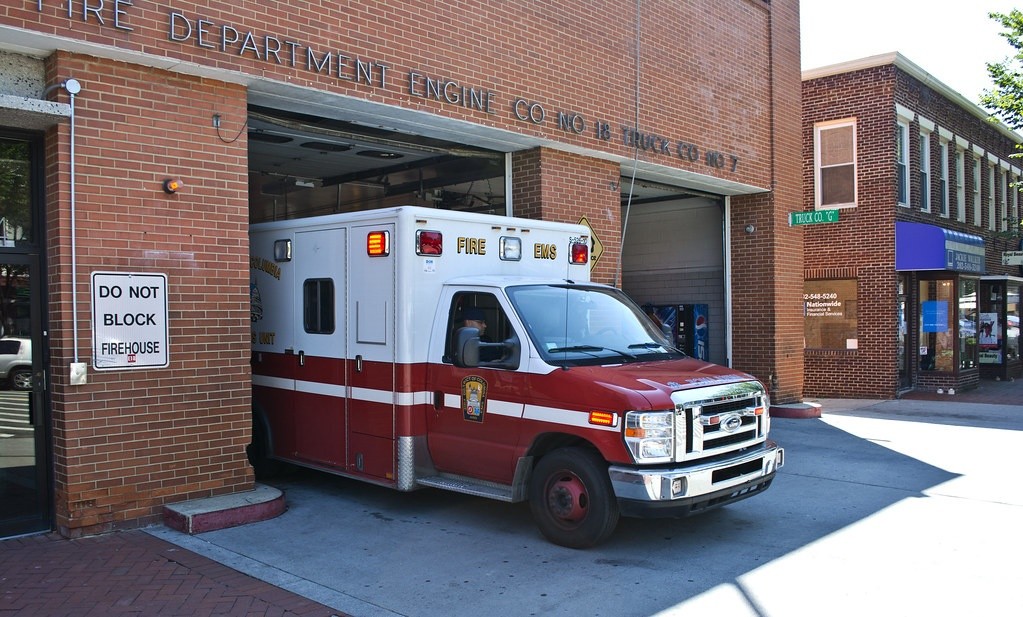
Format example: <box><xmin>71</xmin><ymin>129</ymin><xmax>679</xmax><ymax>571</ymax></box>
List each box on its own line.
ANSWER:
<box><xmin>464</xmin><ymin>310</ymin><xmax>486</xmax><ymax>320</ymax></box>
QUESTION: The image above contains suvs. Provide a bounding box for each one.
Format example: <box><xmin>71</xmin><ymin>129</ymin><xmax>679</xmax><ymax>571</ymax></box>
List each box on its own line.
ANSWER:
<box><xmin>0</xmin><ymin>336</ymin><xmax>33</xmax><ymax>391</ymax></box>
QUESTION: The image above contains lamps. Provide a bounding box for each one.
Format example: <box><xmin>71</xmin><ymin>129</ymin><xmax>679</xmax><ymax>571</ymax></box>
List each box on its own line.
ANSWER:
<box><xmin>294</xmin><ymin>180</ymin><xmax>314</xmax><ymax>188</ymax></box>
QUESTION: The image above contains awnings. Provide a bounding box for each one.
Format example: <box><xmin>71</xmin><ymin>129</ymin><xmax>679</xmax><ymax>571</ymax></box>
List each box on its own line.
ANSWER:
<box><xmin>895</xmin><ymin>220</ymin><xmax>986</xmax><ymax>276</ymax></box>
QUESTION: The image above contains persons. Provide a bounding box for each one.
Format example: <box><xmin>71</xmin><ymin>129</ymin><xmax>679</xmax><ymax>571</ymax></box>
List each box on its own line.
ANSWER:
<box><xmin>463</xmin><ymin>308</ymin><xmax>501</xmax><ymax>363</ymax></box>
<box><xmin>1007</xmin><ymin>320</ymin><xmax>1020</xmax><ymax>360</ymax></box>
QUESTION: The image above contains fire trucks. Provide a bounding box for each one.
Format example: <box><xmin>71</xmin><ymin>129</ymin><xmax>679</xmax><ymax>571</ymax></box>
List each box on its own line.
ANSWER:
<box><xmin>251</xmin><ymin>205</ymin><xmax>786</xmax><ymax>548</ymax></box>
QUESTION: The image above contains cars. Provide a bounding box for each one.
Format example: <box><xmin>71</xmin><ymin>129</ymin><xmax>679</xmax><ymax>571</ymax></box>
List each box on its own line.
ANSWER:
<box><xmin>1007</xmin><ymin>315</ymin><xmax>1020</xmax><ymax>346</ymax></box>
<box><xmin>959</xmin><ymin>292</ymin><xmax>977</xmax><ymax>309</ymax></box>
